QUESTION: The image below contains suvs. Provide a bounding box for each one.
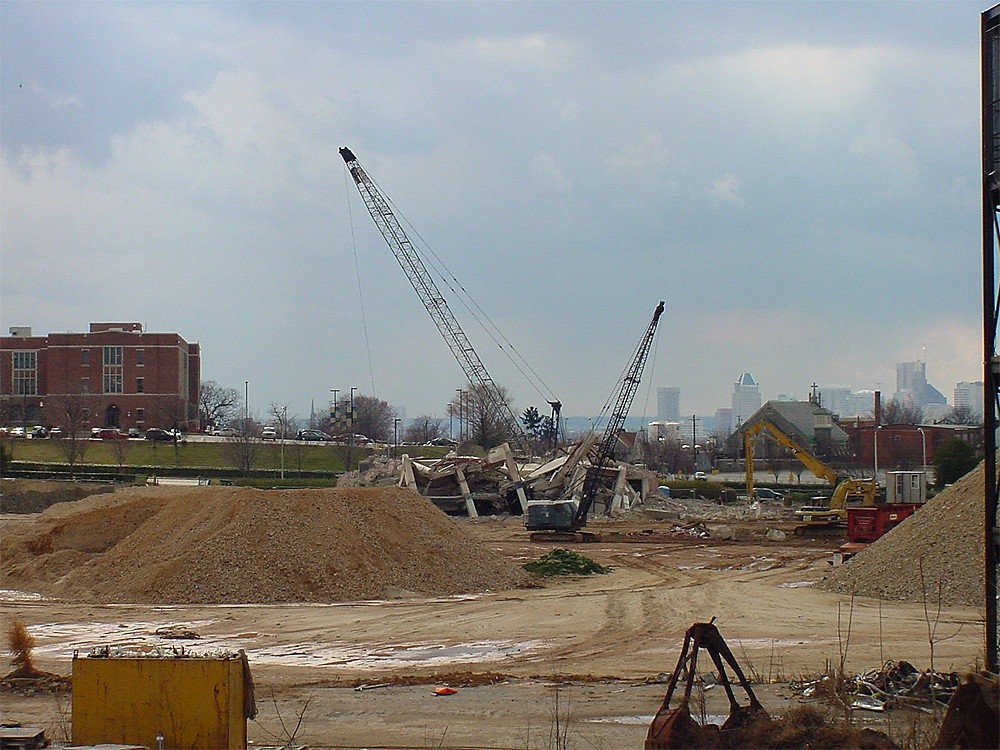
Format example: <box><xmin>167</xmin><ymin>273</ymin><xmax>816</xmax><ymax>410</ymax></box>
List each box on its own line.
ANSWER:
<box><xmin>146</xmin><ymin>428</ymin><xmax>175</xmax><ymax>441</ymax></box>
<box><xmin>753</xmin><ymin>487</ymin><xmax>783</xmax><ymax>499</ymax></box>
<box><xmin>100</xmin><ymin>429</ymin><xmax>129</xmax><ymax>439</ymax></box>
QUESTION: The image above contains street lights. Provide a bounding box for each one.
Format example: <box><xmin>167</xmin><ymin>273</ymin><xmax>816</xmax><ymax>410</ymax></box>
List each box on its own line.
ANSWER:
<box><xmin>447</xmin><ymin>389</ymin><xmax>474</xmax><ymax>440</ymax></box>
<box><xmin>245</xmin><ymin>381</ymin><xmax>248</xmax><ymax>419</ymax></box>
<box><xmin>395</xmin><ymin>418</ymin><xmax>401</xmax><ymax>457</ymax></box>
<box><xmin>918</xmin><ymin>427</ymin><xmax>926</xmax><ymax>470</ymax></box>
<box><xmin>874</xmin><ymin>426</ymin><xmax>881</xmax><ymax>475</ymax></box>
<box><xmin>330</xmin><ymin>387</ymin><xmax>357</xmax><ymax>424</ymax></box>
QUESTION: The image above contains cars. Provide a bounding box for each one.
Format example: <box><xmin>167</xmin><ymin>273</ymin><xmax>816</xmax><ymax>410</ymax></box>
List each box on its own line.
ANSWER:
<box><xmin>296</xmin><ymin>429</ymin><xmax>371</xmax><ymax>444</ymax></box>
<box><xmin>204</xmin><ymin>426</ymin><xmax>240</xmax><ymax>437</ymax></box>
<box><xmin>0</xmin><ymin>426</ymin><xmax>138</xmax><ymax>439</ymax></box>
<box><xmin>170</xmin><ymin>429</ymin><xmax>181</xmax><ymax>439</ymax></box>
<box><xmin>430</xmin><ymin>437</ymin><xmax>457</xmax><ymax>446</ymax></box>
<box><xmin>695</xmin><ymin>472</ymin><xmax>706</xmax><ymax>480</ymax></box>
<box><xmin>261</xmin><ymin>427</ymin><xmax>276</xmax><ymax>440</ymax></box>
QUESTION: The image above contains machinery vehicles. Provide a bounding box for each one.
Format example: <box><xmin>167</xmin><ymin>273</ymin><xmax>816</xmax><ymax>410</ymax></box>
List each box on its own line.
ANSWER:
<box><xmin>338</xmin><ymin>146</ymin><xmax>565</xmax><ymax>461</ymax></box>
<box><xmin>521</xmin><ymin>301</ymin><xmax>665</xmax><ymax>541</ymax></box>
<box><xmin>833</xmin><ymin>501</ymin><xmax>923</xmax><ymax>554</ymax></box>
<box><xmin>744</xmin><ymin>418</ymin><xmax>885</xmax><ymax>536</ymax></box>
